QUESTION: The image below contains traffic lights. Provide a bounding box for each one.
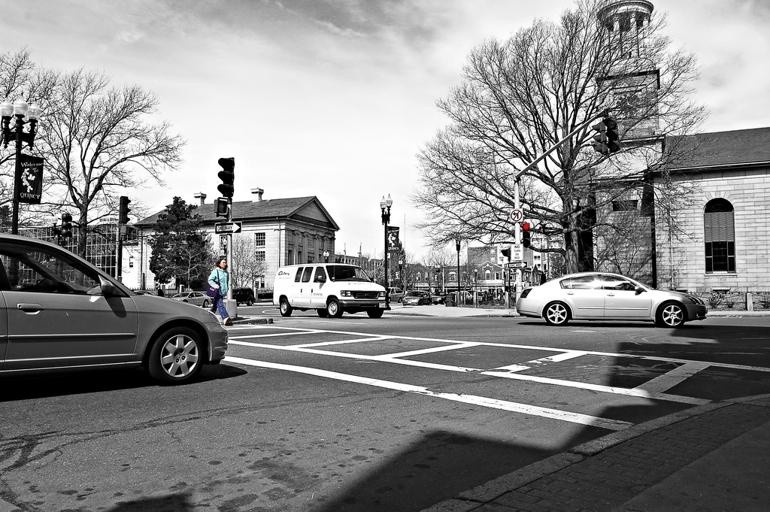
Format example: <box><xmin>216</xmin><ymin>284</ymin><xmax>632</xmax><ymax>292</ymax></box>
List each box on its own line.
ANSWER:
<box><xmin>522</xmin><ymin>222</ymin><xmax>531</xmax><ymax>249</ymax></box>
<box><xmin>591</xmin><ymin>120</ymin><xmax>608</xmax><ymax>156</ymax></box>
<box><xmin>214</xmin><ymin>199</ymin><xmax>229</xmax><ymax>214</ymax></box>
<box><xmin>217</xmin><ymin>155</ymin><xmax>235</xmax><ymax>199</ymax></box>
<box><xmin>604</xmin><ymin>116</ymin><xmax>621</xmax><ymax>153</ymax></box>
<box><xmin>119</xmin><ymin>195</ymin><xmax>132</xmax><ymax>225</ymax></box>
<box><xmin>61</xmin><ymin>212</ymin><xmax>73</xmax><ymax>239</ymax></box>
<box><xmin>501</xmin><ymin>248</ymin><xmax>511</xmax><ymax>257</ymax></box>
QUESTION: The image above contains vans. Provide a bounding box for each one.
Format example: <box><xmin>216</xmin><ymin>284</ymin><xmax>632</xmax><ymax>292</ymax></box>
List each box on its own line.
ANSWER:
<box><xmin>272</xmin><ymin>262</ymin><xmax>389</xmax><ymax>318</ymax></box>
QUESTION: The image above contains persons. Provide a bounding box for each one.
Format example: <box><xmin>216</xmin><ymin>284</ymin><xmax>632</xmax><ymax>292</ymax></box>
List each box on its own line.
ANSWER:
<box><xmin>208</xmin><ymin>259</ymin><xmax>230</xmax><ymax>325</ymax></box>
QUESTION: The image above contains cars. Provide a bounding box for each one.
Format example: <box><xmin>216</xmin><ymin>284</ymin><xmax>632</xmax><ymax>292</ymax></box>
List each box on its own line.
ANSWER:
<box><xmin>173</xmin><ymin>292</ymin><xmax>212</xmax><ymax>308</ymax></box>
<box><xmin>386</xmin><ymin>286</ymin><xmax>493</xmax><ymax>306</ymax></box>
<box><xmin>516</xmin><ymin>272</ymin><xmax>708</xmax><ymax>329</ymax></box>
<box><xmin>0</xmin><ymin>231</ymin><xmax>229</xmax><ymax>386</ymax></box>
<box><xmin>133</xmin><ymin>289</ymin><xmax>152</xmax><ymax>295</ymax></box>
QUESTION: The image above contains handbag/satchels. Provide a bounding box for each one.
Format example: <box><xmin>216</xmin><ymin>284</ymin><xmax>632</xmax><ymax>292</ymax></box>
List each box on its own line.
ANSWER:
<box><xmin>208</xmin><ymin>283</ymin><xmax>219</xmax><ymax>297</ymax></box>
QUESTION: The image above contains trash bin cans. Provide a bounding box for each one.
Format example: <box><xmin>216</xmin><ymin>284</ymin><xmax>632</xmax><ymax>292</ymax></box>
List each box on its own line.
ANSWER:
<box><xmin>446</xmin><ymin>294</ymin><xmax>456</xmax><ymax>307</ymax></box>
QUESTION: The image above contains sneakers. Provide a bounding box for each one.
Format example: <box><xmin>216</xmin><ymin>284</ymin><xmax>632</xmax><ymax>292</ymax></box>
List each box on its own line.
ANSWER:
<box><xmin>223</xmin><ymin>317</ymin><xmax>230</xmax><ymax>324</ymax></box>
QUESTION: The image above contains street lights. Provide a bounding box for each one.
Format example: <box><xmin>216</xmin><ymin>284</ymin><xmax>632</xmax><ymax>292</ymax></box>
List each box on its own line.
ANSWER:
<box><xmin>398</xmin><ymin>261</ymin><xmax>403</xmax><ymax>281</ymax></box>
<box><xmin>379</xmin><ymin>193</ymin><xmax>393</xmax><ymax>310</ymax></box>
<box><xmin>455</xmin><ymin>232</ymin><xmax>461</xmax><ymax>307</ymax></box>
<box><xmin>474</xmin><ymin>269</ymin><xmax>478</xmax><ymax>287</ymax></box>
<box><xmin>437</xmin><ymin>264</ymin><xmax>441</xmax><ymax>287</ymax></box>
<box><xmin>51</xmin><ymin>212</ymin><xmax>58</xmax><ymax>236</ymax></box>
<box><xmin>0</xmin><ymin>98</ymin><xmax>42</xmax><ymax>288</ymax></box>
<box><xmin>223</xmin><ymin>237</ymin><xmax>227</xmax><ymax>257</ymax></box>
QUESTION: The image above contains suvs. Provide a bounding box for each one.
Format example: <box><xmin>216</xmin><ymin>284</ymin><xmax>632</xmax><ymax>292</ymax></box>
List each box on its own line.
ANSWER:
<box><xmin>232</xmin><ymin>287</ymin><xmax>256</xmax><ymax>306</ymax></box>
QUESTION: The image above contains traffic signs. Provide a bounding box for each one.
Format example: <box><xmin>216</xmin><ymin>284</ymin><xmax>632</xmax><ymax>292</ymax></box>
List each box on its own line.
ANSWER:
<box><xmin>507</xmin><ymin>261</ymin><xmax>527</xmax><ymax>270</ymax></box>
<box><xmin>216</xmin><ymin>222</ymin><xmax>242</xmax><ymax>233</ymax></box>
